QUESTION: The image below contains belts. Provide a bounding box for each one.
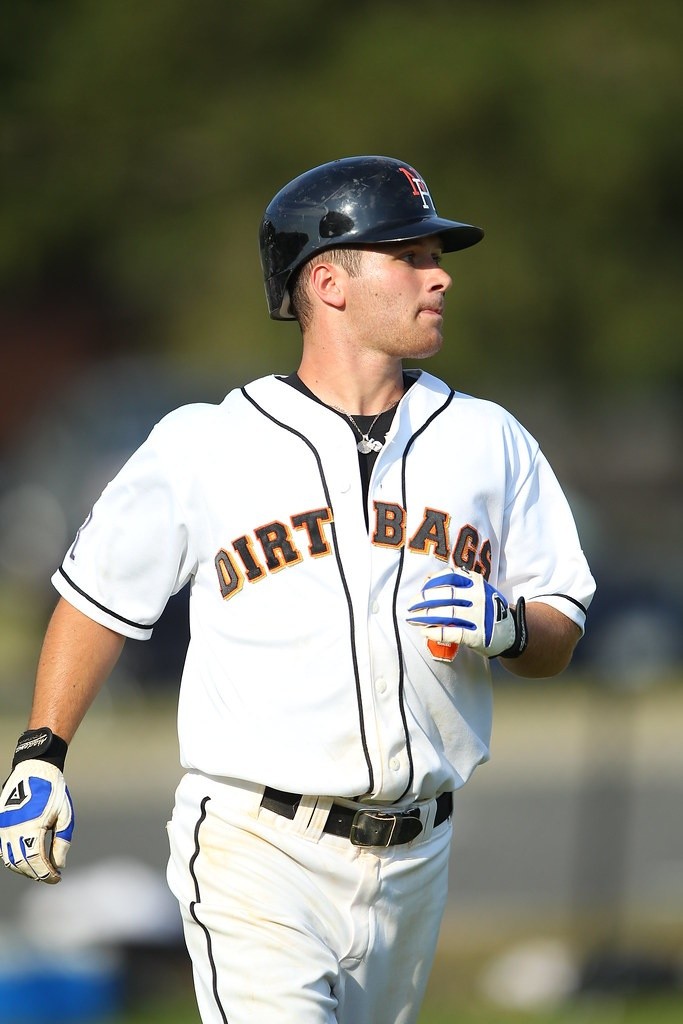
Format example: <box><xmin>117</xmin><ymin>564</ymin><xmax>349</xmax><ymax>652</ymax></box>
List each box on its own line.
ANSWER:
<box><xmin>260</xmin><ymin>786</ymin><xmax>453</xmax><ymax>846</ymax></box>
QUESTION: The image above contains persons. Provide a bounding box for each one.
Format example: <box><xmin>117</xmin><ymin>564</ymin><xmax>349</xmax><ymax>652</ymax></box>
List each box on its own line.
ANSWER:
<box><xmin>0</xmin><ymin>154</ymin><xmax>599</xmax><ymax>1024</ymax></box>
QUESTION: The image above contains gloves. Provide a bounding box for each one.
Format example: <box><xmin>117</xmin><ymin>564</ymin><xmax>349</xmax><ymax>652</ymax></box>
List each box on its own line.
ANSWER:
<box><xmin>404</xmin><ymin>567</ymin><xmax>529</xmax><ymax>658</ymax></box>
<box><xmin>0</xmin><ymin>726</ymin><xmax>75</xmax><ymax>884</ymax></box>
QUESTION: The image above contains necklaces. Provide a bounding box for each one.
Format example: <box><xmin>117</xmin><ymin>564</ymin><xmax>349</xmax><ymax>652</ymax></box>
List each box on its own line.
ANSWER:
<box><xmin>327</xmin><ymin>399</ymin><xmax>400</xmax><ymax>454</ymax></box>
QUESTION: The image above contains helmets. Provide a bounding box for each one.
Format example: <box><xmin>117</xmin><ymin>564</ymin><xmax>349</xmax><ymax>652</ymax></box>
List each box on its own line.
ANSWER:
<box><xmin>260</xmin><ymin>154</ymin><xmax>486</xmax><ymax>321</ymax></box>
